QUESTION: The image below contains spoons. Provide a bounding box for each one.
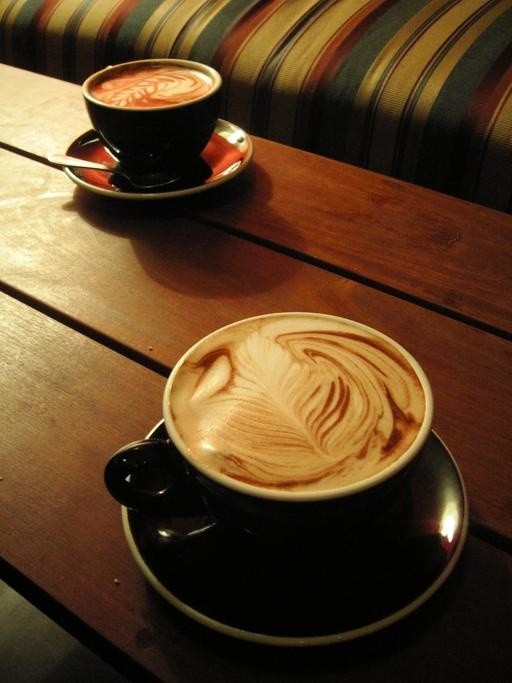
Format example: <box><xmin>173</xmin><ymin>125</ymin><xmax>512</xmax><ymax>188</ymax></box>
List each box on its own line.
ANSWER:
<box><xmin>52</xmin><ymin>152</ymin><xmax>179</xmax><ymax>187</ymax></box>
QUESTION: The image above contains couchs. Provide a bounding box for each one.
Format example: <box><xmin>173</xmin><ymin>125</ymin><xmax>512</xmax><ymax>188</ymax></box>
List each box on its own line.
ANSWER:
<box><xmin>0</xmin><ymin>1</ymin><xmax>512</xmax><ymax>208</ymax></box>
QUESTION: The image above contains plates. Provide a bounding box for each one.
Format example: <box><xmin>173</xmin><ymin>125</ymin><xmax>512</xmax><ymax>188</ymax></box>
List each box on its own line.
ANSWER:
<box><xmin>61</xmin><ymin>117</ymin><xmax>254</xmax><ymax>204</ymax></box>
<box><xmin>122</xmin><ymin>413</ymin><xmax>471</xmax><ymax>651</ymax></box>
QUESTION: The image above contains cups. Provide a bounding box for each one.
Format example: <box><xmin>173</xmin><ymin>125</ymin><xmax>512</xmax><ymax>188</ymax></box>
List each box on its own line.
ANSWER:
<box><xmin>82</xmin><ymin>58</ymin><xmax>223</xmax><ymax>166</ymax></box>
<box><xmin>104</xmin><ymin>310</ymin><xmax>434</xmax><ymax>542</ymax></box>
